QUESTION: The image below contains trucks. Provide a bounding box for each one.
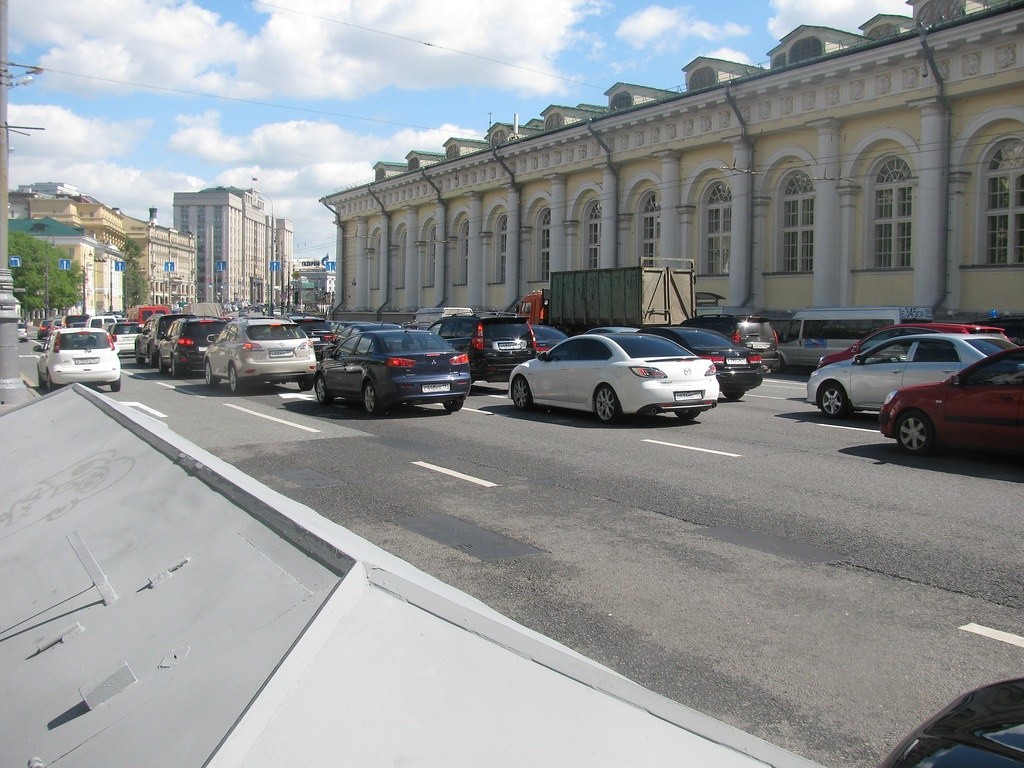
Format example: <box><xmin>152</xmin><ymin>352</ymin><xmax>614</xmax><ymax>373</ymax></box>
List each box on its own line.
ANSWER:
<box><xmin>515</xmin><ymin>256</ymin><xmax>696</xmax><ymax>336</ymax></box>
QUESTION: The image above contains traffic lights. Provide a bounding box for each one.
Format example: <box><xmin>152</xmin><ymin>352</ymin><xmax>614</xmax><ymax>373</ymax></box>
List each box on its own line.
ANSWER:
<box><xmin>96</xmin><ymin>259</ymin><xmax>108</xmax><ymax>263</ymax></box>
<box><xmin>302</xmin><ymin>261</ymin><xmax>321</xmax><ymax>266</ymax></box>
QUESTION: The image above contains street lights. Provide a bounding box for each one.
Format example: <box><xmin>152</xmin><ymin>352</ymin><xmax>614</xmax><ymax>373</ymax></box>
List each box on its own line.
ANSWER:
<box><xmin>0</xmin><ymin>76</ymin><xmax>35</xmax><ymax>405</ymax></box>
<box><xmin>82</xmin><ymin>245</ymin><xmax>95</xmax><ymax>319</ymax></box>
<box><xmin>45</xmin><ymin>232</ymin><xmax>56</xmax><ymax>316</ymax></box>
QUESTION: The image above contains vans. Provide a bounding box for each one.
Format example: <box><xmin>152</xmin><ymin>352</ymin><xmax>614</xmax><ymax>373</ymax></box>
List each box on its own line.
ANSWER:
<box><xmin>772</xmin><ymin>308</ymin><xmax>902</xmax><ymax>375</ymax></box>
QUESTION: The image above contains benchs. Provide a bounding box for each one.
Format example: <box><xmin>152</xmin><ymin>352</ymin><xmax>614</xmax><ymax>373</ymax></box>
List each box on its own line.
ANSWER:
<box><xmin>61</xmin><ymin>337</ymin><xmax>98</xmax><ymax>349</ymax></box>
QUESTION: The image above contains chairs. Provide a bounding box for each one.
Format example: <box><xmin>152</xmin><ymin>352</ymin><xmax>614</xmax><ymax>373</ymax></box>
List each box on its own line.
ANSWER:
<box><xmin>401</xmin><ymin>338</ymin><xmax>414</xmax><ymax>348</ymax></box>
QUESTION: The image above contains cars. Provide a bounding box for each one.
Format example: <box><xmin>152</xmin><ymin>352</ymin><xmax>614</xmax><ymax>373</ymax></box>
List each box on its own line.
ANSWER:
<box><xmin>39</xmin><ymin>301</ymin><xmax>336</xmax><ymax>392</ymax></box>
<box><xmin>38</xmin><ymin>329</ymin><xmax>123</xmax><ymax>394</ymax></box>
<box><xmin>806</xmin><ymin>322</ymin><xmax>1024</xmax><ymax>457</ymax></box>
<box><xmin>315</xmin><ymin>308</ymin><xmax>781</xmax><ymax>426</ymax></box>
<box><xmin>19</xmin><ymin>322</ymin><xmax>29</xmax><ymax>343</ymax></box>
